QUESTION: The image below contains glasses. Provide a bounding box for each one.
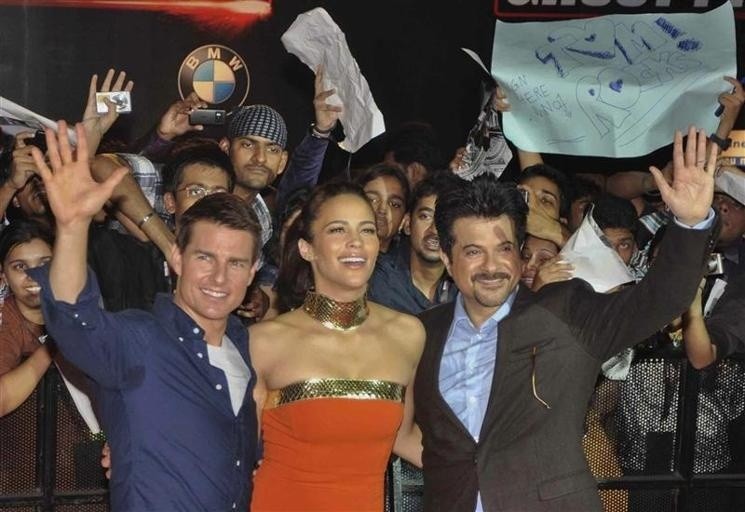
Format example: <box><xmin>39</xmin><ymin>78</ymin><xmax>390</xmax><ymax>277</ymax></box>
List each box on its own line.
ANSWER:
<box><xmin>177</xmin><ymin>183</ymin><xmax>224</xmax><ymax>198</ymax></box>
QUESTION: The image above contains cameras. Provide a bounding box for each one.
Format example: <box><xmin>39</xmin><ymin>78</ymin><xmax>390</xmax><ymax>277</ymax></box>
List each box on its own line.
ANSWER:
<box><xmin>93</xmin><ymin>90</ymin><xmax>131</xmax><ymax>114</ymax></box>
<box><xmin>188</xmin><ymin>108</ymin><xmax>226</xmax><ymax>127</ymax></box>
<box><xmin>21</xmin><ymin>129</ymin><xmax>49</xmax><ymax>155</ymax></box>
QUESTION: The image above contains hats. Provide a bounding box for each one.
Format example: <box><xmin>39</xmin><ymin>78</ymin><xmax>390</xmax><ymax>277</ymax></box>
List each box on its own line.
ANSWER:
<box><xmin>228</xmin><ymin>106</ymin><xmax>288</xmax><ymax>146</ymax></box>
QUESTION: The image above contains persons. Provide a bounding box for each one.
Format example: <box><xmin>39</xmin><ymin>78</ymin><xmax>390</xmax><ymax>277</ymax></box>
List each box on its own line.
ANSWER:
<box><xmin>0</xmin><ymin>64</ymin><xmax>744</xmax><ymax>512</ymax></box>
